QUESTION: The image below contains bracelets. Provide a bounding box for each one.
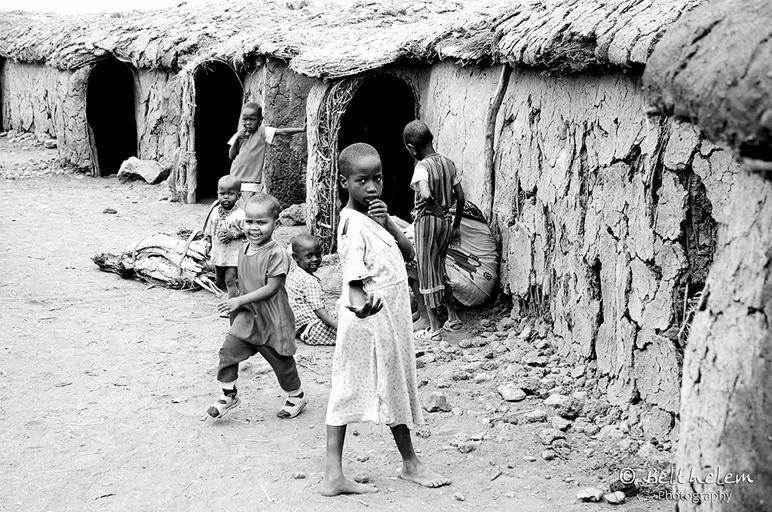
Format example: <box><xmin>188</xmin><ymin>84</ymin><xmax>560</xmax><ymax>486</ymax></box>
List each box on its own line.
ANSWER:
<box><xmin>206</xmin><ymin>243</ymin><xmax>212</xmax><ymax>247</ymax></box>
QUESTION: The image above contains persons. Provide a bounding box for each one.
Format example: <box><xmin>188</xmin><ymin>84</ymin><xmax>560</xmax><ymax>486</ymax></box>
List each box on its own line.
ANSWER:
<box><xmin>227</xmin><ymin>103</ymin><xmax>308</xmax><ymax>194</ymax></box>
<box><xmin>320</xmin><ymin>141</ymin><xmax>452</xmax><ymax>497</ymax></box>
<box><xmin>205</xmin><ymin>175</ymin><xmax>246</xmax><ymax>318</ymax></box>
<box><xmin>207</xmin><ymin>193</ymin><xmax>309</xmax><ymax>418</ymax></box>
<box><xmin>403</xmin><ymin>200</ymin><xmax>500</xmax><ymax>342</ymax></box>
<box><xmin>285</xmin><ymin>233</ymin><xmax>339</xmax><ymax>346</ymax></box>
<box><xmin>403</xmin><ymin>120</ymin><xmax>465</xmax><ymax>341</ymax></box>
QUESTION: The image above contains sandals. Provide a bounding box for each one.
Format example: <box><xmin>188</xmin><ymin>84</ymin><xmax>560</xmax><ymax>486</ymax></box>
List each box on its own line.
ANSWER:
<box><xmin>207</xmin><ymin>389</ymin><xmax>240</xmax><ymax>419</ymax></box>
<box><xmin>277</xmin><ymin>389</ymin><xmax>309</xmax><ymax>419</ymax></box>
<box><xmin>413</xmin><ymin>325</ymin><xmax>442</xmax><ymax>343</ymax></box>
<box><xmin>443</xmin><ymin>318</ymin><xmax>465</xmax><ymax>333</ymax></box>
